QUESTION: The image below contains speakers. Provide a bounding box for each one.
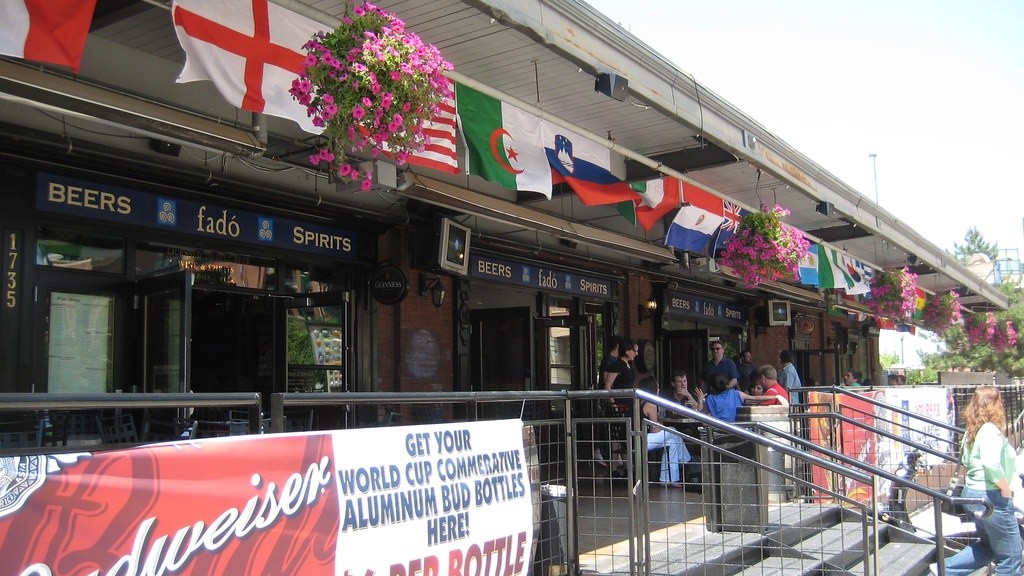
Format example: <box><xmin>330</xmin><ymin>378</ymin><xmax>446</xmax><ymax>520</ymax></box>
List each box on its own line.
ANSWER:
<box><xmin>817</xmin><ymin>201</ymin><xmax>834</xmax><ymax>218</ymax></box>
<box><xmin>724</xmin><ymin>280</ymin><xmax>737</xmax><ymax>287</ymax></box>
<box><xmin>597</xmin><ymin>73</ymin><xmax>629</xmax><ymax>102</ymax></box>
<box><xmin>150</xmin><ymin>139</ymin><xmax>182</xmax><ymax>157</ymax></box>
<box><xmin>907</xmin><ymin>256</ymin><xmax>920</xmax><ymax>266</ymax></box>
<box><xmin>558</xmin><ymin>238</ymin><xmax>578</xmax><ymax>249</ymax></box>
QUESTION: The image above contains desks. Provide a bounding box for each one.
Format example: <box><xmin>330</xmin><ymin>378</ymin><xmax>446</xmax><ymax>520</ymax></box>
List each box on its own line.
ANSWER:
<box><xmin>658</xmin><ymin>416</ymin><xmax>701</xmax><ymax>436</ymax></box>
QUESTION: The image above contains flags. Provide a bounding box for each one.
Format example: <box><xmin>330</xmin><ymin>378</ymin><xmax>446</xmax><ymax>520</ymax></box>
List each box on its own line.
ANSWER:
<box><xmin>0</xmin><ymin>0</ymin><xmax>927</xmax><ymax>329</ymax></box>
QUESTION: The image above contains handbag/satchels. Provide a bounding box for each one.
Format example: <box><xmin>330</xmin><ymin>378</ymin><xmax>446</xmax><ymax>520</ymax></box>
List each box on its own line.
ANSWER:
<box><xmin>940</xmin><ymin>483</ymin><xmax>964</xmax><ymax>516</ymax></box>
<box><xmin>609</xmin><ymin>403</ymin><xmax>632</xmax><ymax>417</ymax></box>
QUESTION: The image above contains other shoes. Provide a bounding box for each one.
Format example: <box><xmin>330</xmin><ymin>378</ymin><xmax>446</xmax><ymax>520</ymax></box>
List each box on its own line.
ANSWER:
<box><xmin>659</xmin><ymin>480</ymin><xmax>682</xmax><ymax>488</ymax></box>
<box><xmin>623</xmin><ymin>473</ymin><xmax>628</xmax><ymax>479</ymax></box>
<box><xmin>929</xmin><ymin>563</ymin><xmax>938</xmax><ymax>576</ymax></box>
<box><xmin>610</xmin><ymin>472</ymin><xmax>622</xmax><ymax>481</ymax></box>
<box><xmin>593</xmin><ymin>450</ymin><xmax>607</xmax><ymax>466</ymax></box>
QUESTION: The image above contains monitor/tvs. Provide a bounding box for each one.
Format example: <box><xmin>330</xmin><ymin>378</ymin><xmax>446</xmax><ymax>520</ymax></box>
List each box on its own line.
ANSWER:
<box><xmin>411</xmin><ymin>217</ymin><xmax>471</xmax><ymax>277</ymax></box>
<box><xmin>756</xmin><ymin>299</ymin><xmax>792</xmax><ymax>327</ymax></box>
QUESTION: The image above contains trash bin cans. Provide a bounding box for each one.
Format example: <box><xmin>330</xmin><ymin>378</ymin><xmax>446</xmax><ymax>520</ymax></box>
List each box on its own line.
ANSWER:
<box><xmin>534</xmin><ymin>484</ymin><xmax>568</xmax><ymax>565</ymax></box>
<box><xmin>697</xmin><ymin>427</ymin><xmax>769</xmax><ymax>533</ymax></box>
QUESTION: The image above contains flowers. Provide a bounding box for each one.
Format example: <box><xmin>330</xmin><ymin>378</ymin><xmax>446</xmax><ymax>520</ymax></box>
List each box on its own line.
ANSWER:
<box><xmin>986</xmin><ymin>321</ymin><xmax>1018</xmax><ymax>351</ymax></box>
<box><xmin>868</xmin><ymin>268</ymin><xmax>919</xmax><ymax>321</ymax></box>
<box><xmin>718</xmin><ymin>206</ymin><xmax>811</xmax><ymax>286</ymax></box>
<box><xmin>922</xmin><ymin>289</ymin><xmax>963</xmax><ymax>334</ymax></box>
<box><xmin>289</xmin><ymin>0</ymin><xmax>454</xmax><ymax>191</ymax></box>
<box><xmin>967</xmin><ymin>309</ymin><xmax>994</xmax><ymax>341</ymax></box>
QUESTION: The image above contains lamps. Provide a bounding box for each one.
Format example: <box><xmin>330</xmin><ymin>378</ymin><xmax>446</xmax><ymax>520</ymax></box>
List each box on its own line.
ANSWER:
<box><xmin>560</xmin><ymin>238</ymin><xmax>579</xmax><ymax>249</ymax></box>
<box><xmin>826</xmin><ymin>336</ymin><xmax>840</xmax><ymax>351</ymax></box>
<box><xmin>417</xmin><ymin>273</ymin><xmax>450</xmax><ymax>312</ymax></box>
<box><xmin>723</xmin><ymin>279</ymin><xmax>736</xmax><ymax>287</ymax></box>
<box><xmin>151</xmin><ymin>141</ymin><xmax>181</xmax><ymax>158</ymax></box>
<box><xmin>638</xmin><ymin>295</ymin><xmax>658</xmax><ymax>325</ymax></box>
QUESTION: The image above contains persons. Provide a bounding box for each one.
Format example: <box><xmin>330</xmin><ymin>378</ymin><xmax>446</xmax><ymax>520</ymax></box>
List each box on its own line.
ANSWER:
<box><xmin>930</xmin><ymin>386</ymin><xmax>1021</xmax><ymax>576</ymax></box>
<box><xmin>592</xmin><ymin>337</ymin><xmax>908</xmax><ymax>487</ymax></box>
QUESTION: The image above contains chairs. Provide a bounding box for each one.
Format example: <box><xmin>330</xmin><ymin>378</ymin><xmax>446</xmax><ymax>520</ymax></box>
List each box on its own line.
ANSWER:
<box><xmin>602</xmin><ymin>400</ymin><xmax>634</xmax><ymax>483</ymax></box>
<box><xmin>0</xmin><ymin>401</ymin><xmax>446</xmax><ymax>458</ymax></box>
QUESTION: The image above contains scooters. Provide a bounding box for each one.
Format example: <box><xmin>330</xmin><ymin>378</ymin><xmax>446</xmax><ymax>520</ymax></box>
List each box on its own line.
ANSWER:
<box><xmin>875</xmin><ymin>444</ymin><xmax>1024</xmax><ymax>550</ymax></box>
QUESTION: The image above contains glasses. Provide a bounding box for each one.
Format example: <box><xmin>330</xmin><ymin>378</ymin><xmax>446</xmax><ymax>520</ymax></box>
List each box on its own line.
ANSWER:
<box><xmin>711</xmin><ymin>347</ymin><xmax>721</xmax><ymax>351</ymax></box>
